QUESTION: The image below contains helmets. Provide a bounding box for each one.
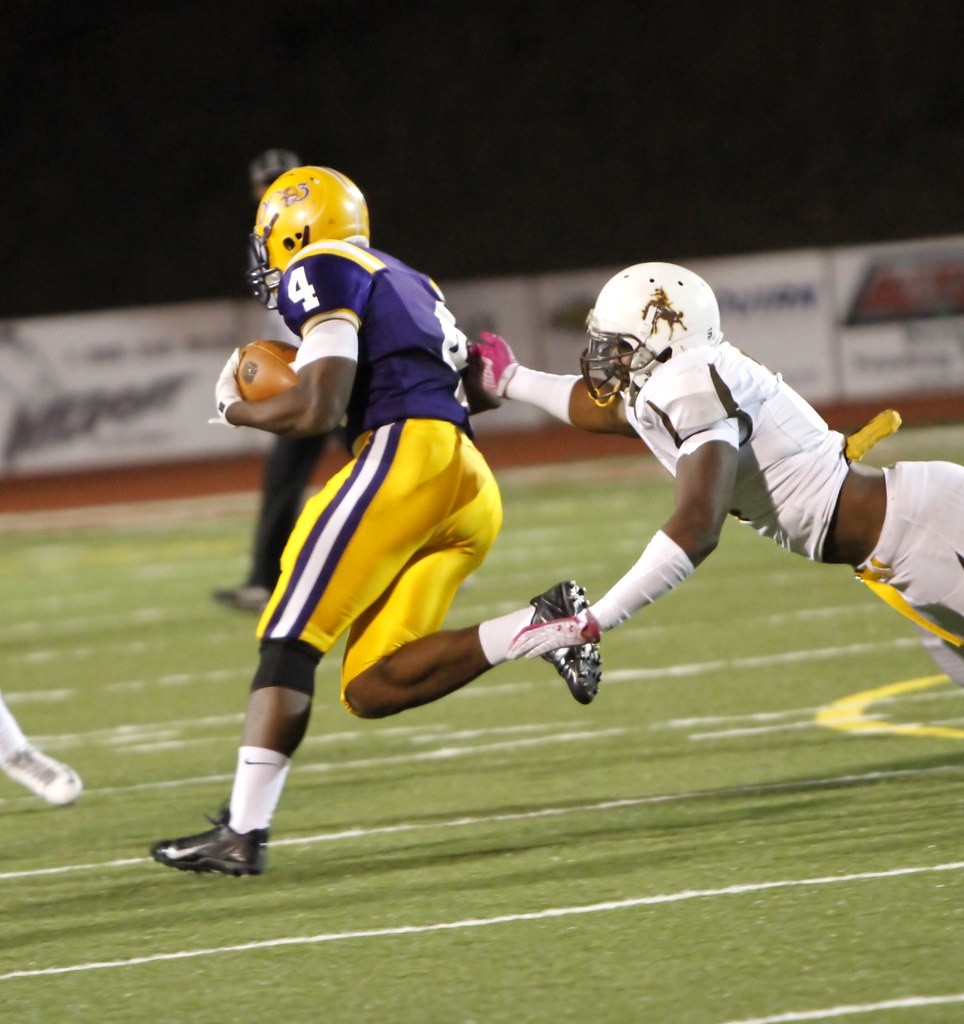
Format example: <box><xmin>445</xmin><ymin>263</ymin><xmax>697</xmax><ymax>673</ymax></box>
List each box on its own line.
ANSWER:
<box><xmin>580</xmin><ymin>262</ymin><xmax>722</xmax><ymax>402</ymax></box>
<box><xmin>247</xmin><ymin>165</ymin><xmax>369</xmax><ymax>308</ymax></box>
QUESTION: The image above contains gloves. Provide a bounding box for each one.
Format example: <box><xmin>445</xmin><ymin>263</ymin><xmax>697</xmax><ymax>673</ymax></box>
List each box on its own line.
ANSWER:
<box><xmin>506</xmin><ymin>607</ymin><xmax>598</xmax><ymax>660</ymax></box>
<box><xmin>209</xmin><ymin>348</ymin><xmax>244</xmax><ymax>428</ymax></box>
<box><xmin>467</xmin><ymin>331</ymin><xmax>517</xmax><ymax>398</ymax></box>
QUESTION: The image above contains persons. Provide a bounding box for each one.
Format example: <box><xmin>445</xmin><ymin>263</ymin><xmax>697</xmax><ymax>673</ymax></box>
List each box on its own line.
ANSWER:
<box><xmin>213</xmin><ymin>148</ymin><xmax>353</xmax><ymax>615</ymax></box>
<box><xmin>465</xmin><ymin>261</ymin><xmax>964</xmax><ymax>694</ymax></box>
<box><xmin>0</xmin><ymin>697</ymin><xmax>81</xmax><ymax>806</ymax></box>
<box><xmin>145</xmin><ymin>166</ymin><xmax>602</xmax><ymax>878</ymax></box>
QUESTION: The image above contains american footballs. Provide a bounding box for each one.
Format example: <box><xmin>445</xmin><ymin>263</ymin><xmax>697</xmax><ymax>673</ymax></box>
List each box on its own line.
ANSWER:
<box><xmin>233</xmin><ymin>338</ymin><xmax>303</xmax><ymax>401</ymax></box>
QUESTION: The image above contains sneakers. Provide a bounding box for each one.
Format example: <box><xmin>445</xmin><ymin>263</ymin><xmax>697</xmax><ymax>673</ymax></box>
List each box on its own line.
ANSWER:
<box><xmin>530</xmin><ymin>580</ymin><xmax>601</xmax><ymax>705</ymax></box>
<box><xmin>3</xmin><ymin>744</ymin><xmax>81</xmax><ymax>804</ymax></box>
<box><xmin>211</xmin><ymin>585</ymin><xmax>269</xmax><ymax>612</ymax></box>
<box><xmin>152</xmin><ymin>809</ymin><xmax>269</xmax><ymax>877</ymax></box>
